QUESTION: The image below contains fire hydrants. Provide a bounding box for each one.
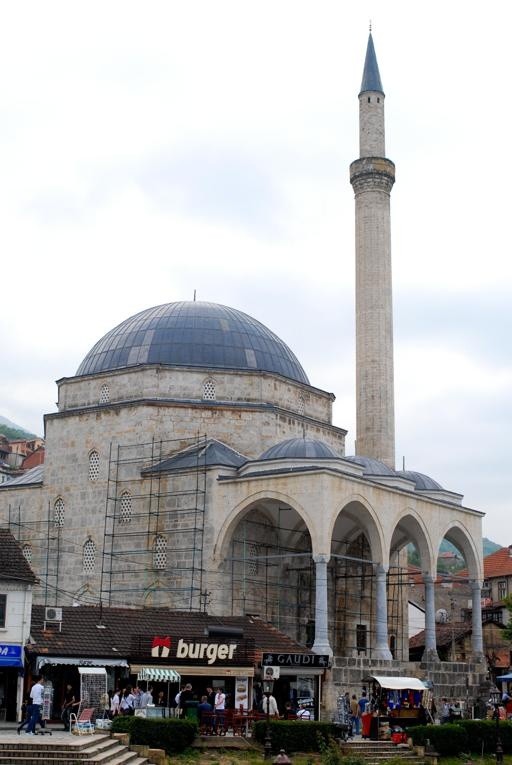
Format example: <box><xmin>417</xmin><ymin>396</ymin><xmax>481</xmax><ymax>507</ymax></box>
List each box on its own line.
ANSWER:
<box><xmin>270</xmin><ymin>749</ymin><xmax>291</xmax><ymax>765</ymax></box>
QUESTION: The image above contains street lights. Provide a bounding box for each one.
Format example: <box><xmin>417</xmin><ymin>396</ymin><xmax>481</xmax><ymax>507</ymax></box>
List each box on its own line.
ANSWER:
<box><xmin>259</xmin><ymin>671</ymin><xmax>275</xmax><ymax>753</ymax></box>
<box><xmin>490</xmin><ymin>686</ymin><xmax>503</xmax><ymax>765</ymax></box>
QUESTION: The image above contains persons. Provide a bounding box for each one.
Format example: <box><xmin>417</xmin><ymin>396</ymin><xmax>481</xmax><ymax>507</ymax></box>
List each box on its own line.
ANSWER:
<box><xmin>61</xmin><ymin>684</ymin><xmax>76</xmax><ymax>731</ymax></box>
<box><xmin>297</xmin><ymin>704</ymin><xmax>309</xmax><ymax>720</ymax></box>
<box><xmin>440</xmin><ymin>694</ymin><xmax>511</xmax><ymax>720</ymax></box>
<box><xmin>341</xmin><ymin>689</ymin><xmax>425</xmax><ymax>736</ymax></box>
<box><xmin>99</xmin><ymin>683</ymin><xmax>162</xmax><ymax>729</ymax></box>
<box><xmin>17</xmin><ymin>686</ymin><xmax>39</xmax><ymax>736</ymax></box>
<box><xmin>25</xmin><ymin>674</ymin><xmax>46</xmax><ymax>736</ymax></box>
<box><xmin>175</xmin><ymin>683</ymin><xmax>226</xmax><ymax>735</ymax></box>
<box><xmin>263</xmin><ymin>693</ymin><xmax>279</xmax><ymax>720</ymax></box>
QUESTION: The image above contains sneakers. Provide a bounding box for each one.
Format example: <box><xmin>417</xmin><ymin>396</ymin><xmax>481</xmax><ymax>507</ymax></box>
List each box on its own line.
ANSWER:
<box><xmin>17</xmin><ymin>728</ymin><xmax>36</xmax><ymax>736</ymax></box>
